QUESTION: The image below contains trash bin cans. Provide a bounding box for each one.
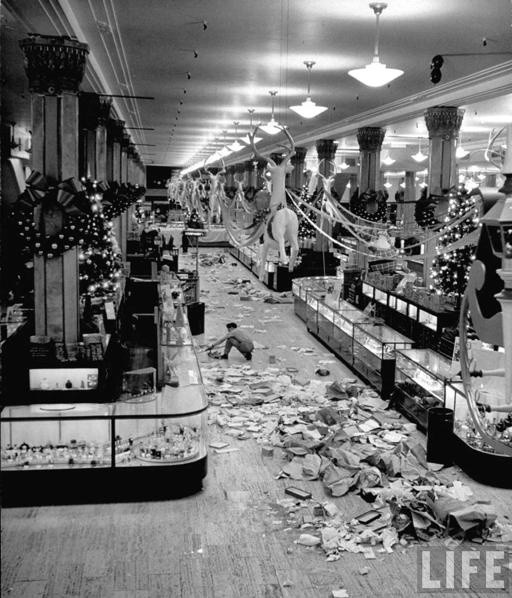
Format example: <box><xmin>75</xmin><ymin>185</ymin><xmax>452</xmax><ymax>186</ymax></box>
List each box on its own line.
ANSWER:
<box><xmin>186</xmin><ymin>302</ymin><xmax>205</xmax><ymax>335</ymax></box>
<box><xmin>426</xmin><ymin>407</ymin><xmax>454</xmax><ymax>468</ymax></box>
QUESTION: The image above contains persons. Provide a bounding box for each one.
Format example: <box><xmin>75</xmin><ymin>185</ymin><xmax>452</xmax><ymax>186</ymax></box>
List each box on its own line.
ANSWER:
<box><xmin>206</xmin><ymin>321</ymin><xmax>254</xmax><ymax>360</ymax></box>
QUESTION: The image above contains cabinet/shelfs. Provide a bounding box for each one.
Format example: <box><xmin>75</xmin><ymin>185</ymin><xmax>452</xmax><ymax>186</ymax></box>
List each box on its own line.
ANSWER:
<box><xmin>0</xmin><ymin>226</ymin><xmax>225</xmax><ymax>510</ymax></box>
<box><xmin>233</xmin><ymin>239</ymin><xmax>511</xmax><ymax>487</ymax></box>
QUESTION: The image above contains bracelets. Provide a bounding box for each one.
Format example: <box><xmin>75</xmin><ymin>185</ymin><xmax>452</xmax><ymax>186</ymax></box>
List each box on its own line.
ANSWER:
<box><xmin>212</xmin><ymin>343</ymin><xmax>215</xmax><ymax>348</ymax></box>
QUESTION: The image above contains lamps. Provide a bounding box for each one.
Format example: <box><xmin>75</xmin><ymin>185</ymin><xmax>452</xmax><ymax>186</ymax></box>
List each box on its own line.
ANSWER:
<box><xmin>381</xmin><ymin>136</ymin><xmax>468</xmax><ymax>165</ymax></box>
<box><xmin>219</xmin><ymin>3</ymin><xmax>406</xmax><ymax>157</ymax></box>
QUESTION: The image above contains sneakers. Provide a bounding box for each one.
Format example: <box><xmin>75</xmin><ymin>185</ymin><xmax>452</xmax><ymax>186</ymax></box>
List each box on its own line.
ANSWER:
<box><xmin>215</xmin><ymin>354</ymin><xmax>228</xmax><ymax>360</ymax></box>
<box><xmin>244</xmin><ymin>352</ymin><xmax>252</xmax><ymax>361</ymax></box>
